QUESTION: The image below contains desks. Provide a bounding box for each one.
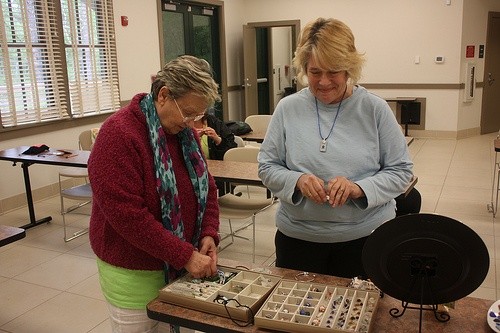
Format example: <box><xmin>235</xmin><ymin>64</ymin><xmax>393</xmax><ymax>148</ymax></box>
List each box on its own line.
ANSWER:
<box><xmin>236</xmin><ymin>130</ymin><xmax>415</xmax><ymax>202</ymax></box>
<box><xmin>381</xmin><ymin>97</ymin><xmax>417</xmax><ymax>137</ymax></box>
<box><xmin>0</xmin><ymin>145</ymin><xmax>269</xmax><ymax>230</ymax></box>
<box><xmin>0</xmin><ymin>225</ymin><xmax>26</xmax><ymax>248</ymax></box>
<box><xmin>146</xmin><ymin>257</ymin><xmax>500</xmax><ymax>333</ymax></box>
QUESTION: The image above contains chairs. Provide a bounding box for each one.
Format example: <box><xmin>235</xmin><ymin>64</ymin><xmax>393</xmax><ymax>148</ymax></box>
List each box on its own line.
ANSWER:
<box><xmin>393</xmin><ymin>186</ymin><xmax>423</xmax><ymax>218</ymax></box>
<box><xmin>58</xmin><ymin>128</ymin><xmax>102</xmax><ymax>216</ymax></box>
<box><xmin>217</xmin><ymin>147</ymin><xmax>276</xmax><ymax>265</ymax></box>
<box><xmin>241</xmin><ymin>114</ymin><xmax>274</xmax><ymax>199</ymax></box>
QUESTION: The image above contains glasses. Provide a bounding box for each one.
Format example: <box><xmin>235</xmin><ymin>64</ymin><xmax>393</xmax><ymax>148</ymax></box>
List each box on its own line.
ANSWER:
<box><xmin>169</xmin><ymin>90</ymin><xmax>204</xmax><ymax>122</ymax></box>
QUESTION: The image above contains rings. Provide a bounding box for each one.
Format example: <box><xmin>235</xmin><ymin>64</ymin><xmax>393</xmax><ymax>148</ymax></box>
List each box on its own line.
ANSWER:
<box><xmin>308</xmin><ymin>193</ymin><xmax>311</xmax><ymax>198</ymax></box>
<box><xmin>341</xmin><ymin>189</ymin><xmax>344</xmax><ymax>191</ymax></box>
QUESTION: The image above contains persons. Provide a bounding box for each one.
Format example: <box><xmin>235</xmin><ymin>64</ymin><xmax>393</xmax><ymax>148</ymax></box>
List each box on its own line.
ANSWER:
<box><xmin>87</xmin><ymin>56</ymin><xmax>221</xmax><ymax>333</ymax></box>
<box><xmin>258</xmin><ymin>20</ymin><xmax>414</xmax><ymax>279</ymax></box>
<box><xmin>201</xmin><ymin>112</ymin><xmax>237</xmax><ymax>189</ymax></box>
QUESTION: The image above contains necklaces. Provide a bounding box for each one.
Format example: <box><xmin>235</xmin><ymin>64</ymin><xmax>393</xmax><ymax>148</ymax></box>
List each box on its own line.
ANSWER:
<box><xmin>316</xmin><ymin>84</ymin><xmax>347</xmax><ymax>151</ymax></box>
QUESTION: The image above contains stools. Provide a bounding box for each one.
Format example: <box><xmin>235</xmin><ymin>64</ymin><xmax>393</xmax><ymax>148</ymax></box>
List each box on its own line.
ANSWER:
<box><xmin>59</xmin><ymin>180</ymin><xmax>93</xmax><ymax>243</ymax></box>
